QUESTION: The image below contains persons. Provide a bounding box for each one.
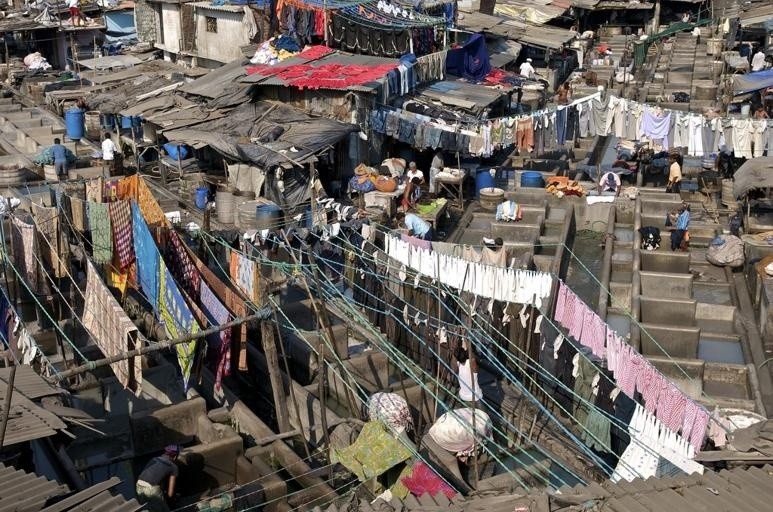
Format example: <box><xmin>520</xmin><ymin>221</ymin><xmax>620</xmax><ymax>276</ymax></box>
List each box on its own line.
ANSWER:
<box><xmin>681</xmin><ymin>10</ymin><xmax>694</xmax><ymax>23</ymax></box>
<box><xmin>664</xmin><ymin>154</ymin><xmax>682</xmax><ymax>193</ymax></box>
<box><xmin>135</xmin><ymin>444</ymin><xmax>182</xmax><ymax>512</ymax></box>
<box><xmin>557</xmin><ymin>81</ymin><xmax>570</xmax><ymax>107</ymax></box>
<box><xmin>48</xmin><ymin>137</ymin><xmax>70</xmax><ymax>176</ymax></box>
<box><xmin>406</xmin><ymin>162</ymin><xmax>425</xmax><ymax>200</ymax></box>
<box><xmin>428</xmin><ymin>147</ymin><xmax>448</xmax><ymax>198</ymax></box>
<box><xmin>597</xmin><ymin>171</ymin><xmax>622</xmax><ymax>199</ymax></box>
<box><xmin>68</xmin><ymin>0</ymin><xmax>81</xmax><ymax>28</ymax></box>
<box><xmin>714</xmin><ymin>153</ymin><xmax>736</xmax><ymax>179</ymax></box>
<box><xmin>752</xmin><ymin>104</ymin><xmax>770</xmax><ymax>118</ymax></box>
<box><xmin>751</xmin><ymin>48</ymin><xmax>767</xmax><ymax>71</ymax></box>
<box><xmin>581</xmin><ymin>29</ymin><xmax>597</xmax><ymax>38</ymax></box>
<box><xmin>493</xmin><ymin>238</ymin><xmax>505</xmax><ymax>252</ymax></box>
<box><xmin>669</xmin><ymin>201</ymin><xmax>690</xmax><ymax>252</ymax></box>
<box><xmin>452</xmin><ymin>325</ymin><xmax>489</xmax><ymax>412</ymax></box>
<box><xmin>101</xmin><ymin>131</ymin><xmax>117</xmax><ymax>168</ymax></box>
<box><xmin>394</xmin><ymin>211</ymin><xmax>434</xmax><ymax>242</ymax></box>
<box><xmin>519</xmin><ymin>56</ymin><xmax>536</xmax><ymax>79</ymax></box>
<box><xmin>401</xmin><ymin>176</ymin><xmax>421</xmax><ymax>213</ymax></box>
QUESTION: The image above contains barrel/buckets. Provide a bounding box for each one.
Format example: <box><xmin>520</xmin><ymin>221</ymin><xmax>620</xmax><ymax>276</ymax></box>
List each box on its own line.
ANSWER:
<box><xmin>65</xmin><ymin>109</ymin><xmax>155</xmax><ymax>142</ymax></box>
<box><xmin>699</xmin><ymin>171</ymin><xmax>722</xmax><ymax>193</ymax></box>
<box><xmin>475</xmin><ymin>168</ymin><xmax>496</xmax><ymax>200</ymax></box>
<box><xmin>695</xmin><ymin>37</ymin><xmax>740</xmax><ymax>99</ymax></box>
<box><xmin>521</xmin><ymin>173</ymin><xmax>542</xmax><ymax>188</ymax></box>
<box><xmin>196</xmin><ymin>187</ymin><xmax>285</xmax><ymax>231</ymax></box>
<box><xmin>721</xmin><ymin>178</ymin><xmax>744</xmax><ymax>207</ymax></box>
<box><xmin>478</xmin><ymin>188</ymin><xmax>504</xmax><ymax>209</ymax></box>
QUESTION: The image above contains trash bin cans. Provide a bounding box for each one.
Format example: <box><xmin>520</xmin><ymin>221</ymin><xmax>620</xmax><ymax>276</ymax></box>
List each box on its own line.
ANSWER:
<box><xmin>66</xmin><ymin>109</ymin><xmax>116</xmax><ymax>139</ymax></box>
<box><xmin>196</xmin><ymin>187</ymin><xmax>209</xmax><ymax>209</ymax></box>
<box><xmin>256</xmin><ymin>205</ymin><xmax>285</xmax><ymax>231</ymax></box>
<box><xmin>520</xmin><ymin>171</ymin><xmax>543</xmax><ymax>188</ymax></box>
<box><xmin>476</xmin><ymin>166</ymin><xmax>509</xmax><ymax>199</ymax></box>
